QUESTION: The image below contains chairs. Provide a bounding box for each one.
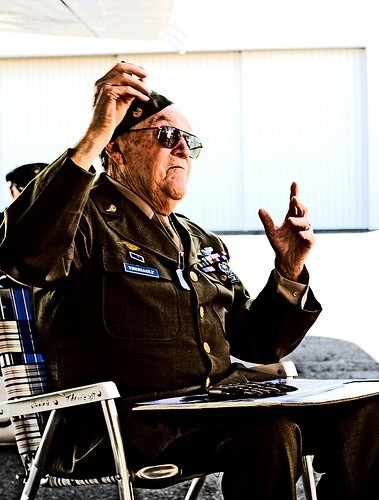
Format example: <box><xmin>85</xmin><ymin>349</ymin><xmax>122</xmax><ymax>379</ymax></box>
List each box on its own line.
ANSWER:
<box><xmin>0</xmin><ymin>273</ymin><xmax>318</xmax><ymax>500</ymax></box>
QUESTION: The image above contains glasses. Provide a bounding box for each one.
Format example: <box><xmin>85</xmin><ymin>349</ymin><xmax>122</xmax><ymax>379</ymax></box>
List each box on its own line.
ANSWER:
<box><xmin>126</xmin><ymin>126</ymin><xmax>203</xmax><ymax>160</ymax></box>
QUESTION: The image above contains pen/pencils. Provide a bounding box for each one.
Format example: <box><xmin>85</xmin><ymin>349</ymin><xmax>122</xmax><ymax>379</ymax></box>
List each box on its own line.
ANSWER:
<box><xmin>179</xmin><ymin>395</ymin><xmax>208</xmax><ymax>403</ymax></box>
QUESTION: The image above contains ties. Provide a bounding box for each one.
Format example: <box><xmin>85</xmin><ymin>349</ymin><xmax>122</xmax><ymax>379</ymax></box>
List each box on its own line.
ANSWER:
<box><xmin>153</xmin><ymin>213</ymin><xmax>184</xmax><ymax>255</ymax></box>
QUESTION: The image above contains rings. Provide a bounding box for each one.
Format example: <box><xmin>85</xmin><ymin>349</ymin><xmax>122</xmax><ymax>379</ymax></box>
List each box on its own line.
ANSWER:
<box><xmin>306</xmin><ymin>224</ymin><xmax>311</xmax><ymax>230</ymax></box>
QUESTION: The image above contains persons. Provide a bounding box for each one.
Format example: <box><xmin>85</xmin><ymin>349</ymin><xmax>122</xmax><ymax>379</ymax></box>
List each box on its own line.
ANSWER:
<box><xmin>0</xmin><ymin>63</ymin><xmax>379</xmax><ymax>500</ymax></box>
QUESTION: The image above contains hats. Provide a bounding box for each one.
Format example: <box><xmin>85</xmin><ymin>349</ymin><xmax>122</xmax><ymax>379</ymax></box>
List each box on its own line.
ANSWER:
<box><xmin>6</xmin><ymin>162</ymin><xmax>50</xmax><ymax>186</ymax></box>
<box><xmin>100</xmin><ymin>90</ymin><xmax>175</xmax><ymax>159</ymax></box>
